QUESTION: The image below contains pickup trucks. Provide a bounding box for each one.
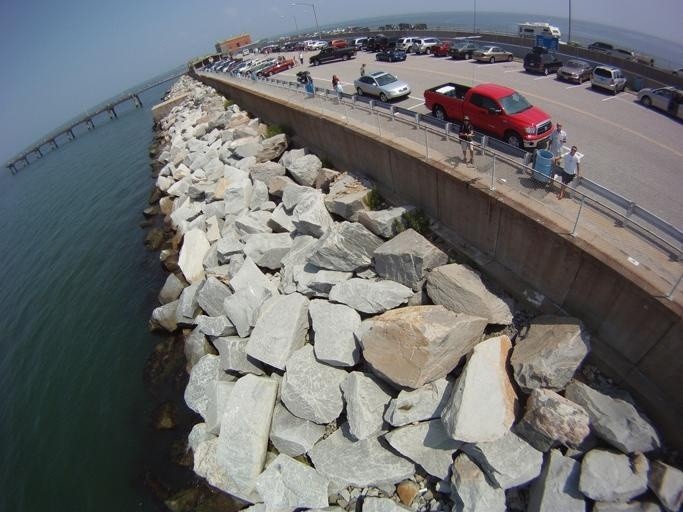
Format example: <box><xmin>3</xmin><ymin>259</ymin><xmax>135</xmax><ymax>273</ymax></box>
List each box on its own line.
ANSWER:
<box><xmin>423</xmin><ymin>81</ymin><xmax>555</xmax><ymax>150</ymax></box>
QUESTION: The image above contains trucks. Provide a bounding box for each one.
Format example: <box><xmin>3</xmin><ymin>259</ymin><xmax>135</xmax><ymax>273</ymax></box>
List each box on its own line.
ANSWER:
<box><xmin>518</xmin><ymin>22</ymin><xmax>561</xmax><ymax>41</ymax></box>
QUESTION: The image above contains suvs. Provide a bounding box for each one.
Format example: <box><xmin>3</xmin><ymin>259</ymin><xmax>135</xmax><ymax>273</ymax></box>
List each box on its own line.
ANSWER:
<box><xmin>523</xmin><ymin>46</ymin><xmax>563</xmax><ymax>76</ymax></box>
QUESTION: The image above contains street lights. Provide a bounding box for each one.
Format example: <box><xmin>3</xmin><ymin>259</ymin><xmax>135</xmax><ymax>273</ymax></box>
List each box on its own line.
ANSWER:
<box><xmin>289</xmin><ymin>3</ymin><xmax>321</xmax><ymax>39</ymax></box>
<box><xmin>282</xmin><ymin>15</ymin><xmax>298</xmax><ymax>35</ymax></box>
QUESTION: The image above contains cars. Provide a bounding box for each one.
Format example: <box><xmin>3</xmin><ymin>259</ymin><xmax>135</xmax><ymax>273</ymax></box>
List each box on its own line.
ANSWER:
<box><xmin>350</xmin><ymin>34</ymin><xmax>514</xmax><ymax>64</ymax></box>
<box><xmin>672</xmin><ymin>68</ymin><xmax>683</xmax><ymax>80</ymax></box>
<box><xmin>322</xmin><ymin>23</ymin><xmax>429</xmax><ymax>33</ymax></box>
<box><xmin>354</xmin><ymin>71</ymin><xmax>412</xmax><ymax>103</ymax></box>
<box><xmin>203</xmin><ymin>39</ymin><xmax>358</xmax><ymax>78</ymax></box>
<box><xmin>637</xmin><ymin>86</ymin><xmax>683</xmax><ymax>122</ymax></box>
<box><xmin>556</xmin><ymin>59</ymin><xmax>593</xmax><ymax>86</ymax></box>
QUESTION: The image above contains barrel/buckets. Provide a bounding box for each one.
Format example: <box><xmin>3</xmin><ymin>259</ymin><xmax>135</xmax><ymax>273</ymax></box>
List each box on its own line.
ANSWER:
<box><xmin>534</xmin><ymin>149</ymin><xmax>554</xmax><ymax>184</ymax></box>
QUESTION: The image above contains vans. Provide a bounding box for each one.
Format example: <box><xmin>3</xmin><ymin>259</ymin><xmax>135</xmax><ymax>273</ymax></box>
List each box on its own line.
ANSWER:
<box><xmin>588</xmin><ymin>42</ymin><xmax>614</xmax><ymax>54</ymax></box>
<box><xmin>589</xmin><ymin>65</ymin><xmax>627</xmax><ymax>96</ymax></box>
<box><xmin>606</xmin><ymin>49</ymin><xmax>635</xmax><ymax>61</ymax></box>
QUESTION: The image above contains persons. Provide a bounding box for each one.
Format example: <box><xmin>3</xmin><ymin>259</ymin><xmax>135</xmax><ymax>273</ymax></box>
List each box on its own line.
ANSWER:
<box><xmin>553</xmin><ymin>145</ymin><xmax>581</xmax><ymax>199</ymax></box>
<box><xmin>360</xmin><ymin>63</ymin><xmax>367</xmax><ymax>77</ymax></box>
<box><xmin>547</xmin><ymin>121</ymin><xmax>567</xmax><ymax>167</ymax></box>
<box><xmin>458</xmin><ymin>115</ymin><xmax>475</xmax><ymax>164</ymax></box>
<box><xmin>330</xmin><ymin>74</ymin><xmax>343</xmax><ymax>98</ymax></box>
